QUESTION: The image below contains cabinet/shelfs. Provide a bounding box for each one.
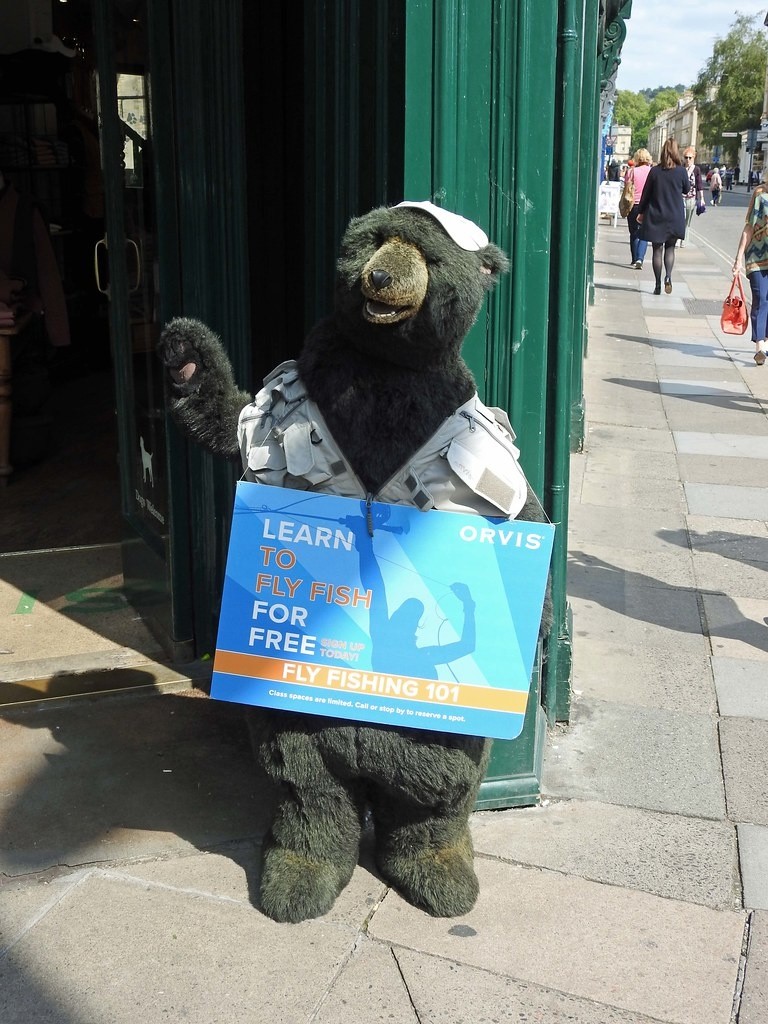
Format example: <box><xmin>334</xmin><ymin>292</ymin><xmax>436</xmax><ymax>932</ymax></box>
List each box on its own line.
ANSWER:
<box><xmin>0</xmin><ymin>48</ymin><xmax>101</xmax><ymax>382</ymax></box>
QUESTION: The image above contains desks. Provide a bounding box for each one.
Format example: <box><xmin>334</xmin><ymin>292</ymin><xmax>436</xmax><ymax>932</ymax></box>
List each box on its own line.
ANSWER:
<box><xmin>0</xmin><ymin>312</ymin><xmax>39</xmax><ymax>485</ymax></box>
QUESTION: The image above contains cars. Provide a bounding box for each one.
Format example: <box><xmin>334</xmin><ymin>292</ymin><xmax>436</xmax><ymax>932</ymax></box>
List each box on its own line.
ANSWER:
<box><xmin>705</xmin><ymin>170</ymin><xmax>728</xmax><ymax>187</ymax></box>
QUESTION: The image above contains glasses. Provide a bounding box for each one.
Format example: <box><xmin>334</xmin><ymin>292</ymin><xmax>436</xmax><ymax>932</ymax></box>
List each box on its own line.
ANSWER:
<box><xmin>685</xmin><ymin>157</ymin><xmax>693</xmax><ymax>159</ymax></box>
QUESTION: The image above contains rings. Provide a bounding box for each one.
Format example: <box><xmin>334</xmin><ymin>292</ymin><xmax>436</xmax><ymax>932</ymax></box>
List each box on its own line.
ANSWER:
<box><xmin>732</xmin><ymin>268</ymin><xmax>735</xmax><ymax>271</ymax></box>
<box><xmin>704</xmin><ymin>203</ymin><xmax>705</xmax><ymax>204</ymax></box>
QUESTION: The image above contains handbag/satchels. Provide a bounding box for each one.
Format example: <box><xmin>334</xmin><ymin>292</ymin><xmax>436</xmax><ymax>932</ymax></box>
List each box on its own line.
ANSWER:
<box><xmin>720</xmin><ymin>271</ymin><xmax>749</xmax><ymax>335</ymax></box>
<box><xmin>619</xmin><ymin>167</ymin><xmax>635</xmax><ymax>219</ymax></box>
<box><xmin>717</xmin><ymin>190</ymin><xmax>722</xmax><ymax>204</ymax></box>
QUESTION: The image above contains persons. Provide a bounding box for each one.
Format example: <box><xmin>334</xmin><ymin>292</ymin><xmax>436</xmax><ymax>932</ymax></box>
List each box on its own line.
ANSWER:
<box><xmin>123</xmin><ymin>192</ymin><xmax>137</xmax><ymax>232</ymax></box>
<box><xmin>619</xmin><ymin>166</ymin><xmax>629</xmax><ymax>179</ymax></box>
<box><xmin>625</xmin><ymin>147</ymin><xmax>653</xmax><ymax>269</ymax></box>
<box><xmin>674</xmin><ymin>147</ymin><xmax>705</xmax><ymax>249</ymax></box>
<box><xmin>701</xmin><ymin>162</ymin><xmax>762</xmax><ymax>191</ymax></box>
<box><xmin>636</xmin><ymin>137</ymin><xmax>690</xmax><ymax>296</ymax></box>
<box><xmin>732</xmin><ymin>160</ymin><xmax>768</xmax><ymax>365</ymax></box>
<box><xmin>709</xmin><ymin>167</ymin><xmax>722</xmax><ymax>206</ymax></box>
<box><xmin>650</xmin><ymin>158</ymin><xmax>662</xmax><ymax>170</ymax></box>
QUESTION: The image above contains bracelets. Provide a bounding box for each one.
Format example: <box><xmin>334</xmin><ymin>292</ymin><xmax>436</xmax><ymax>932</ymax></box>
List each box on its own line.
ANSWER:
<box><xmin>700</xmin><ymin>196</ymin><xmax>704</xmax><ymax>198</ymax></box>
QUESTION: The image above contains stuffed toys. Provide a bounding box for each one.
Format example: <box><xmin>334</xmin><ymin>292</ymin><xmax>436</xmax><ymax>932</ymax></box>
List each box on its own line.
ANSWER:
<box><xmin>155</xmin><ymin>203</ymin><xmax>555</xmax><ymax>925</ymax></box>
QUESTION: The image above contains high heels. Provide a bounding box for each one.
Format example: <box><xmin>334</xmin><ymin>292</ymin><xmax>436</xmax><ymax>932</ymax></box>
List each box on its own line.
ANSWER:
<box><xmin>665</xmin><ymin>276</ymin><xmax>672</xmax><ymax>295</ymax></box>
<box><xmin>654</xmin><ymin>284</ymin><xmax>661</xmax><ymax>295</ymax></box>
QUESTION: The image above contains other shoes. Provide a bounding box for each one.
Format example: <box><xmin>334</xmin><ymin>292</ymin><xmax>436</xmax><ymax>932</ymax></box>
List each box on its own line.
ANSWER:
<box><xmin>680</xmin><ymin>240</ymin><xmax>685</xmax><ymax>248</ymax></box>
<box><xmin>710</xmin><ymin>200</ymin><xmax>714</xmax><ymax>206</ymax></box>
<box><xmin>754</xmin><ymin>351</ymin><xmax>765</xmax><ymax>367</ymax></box>
<box><xmin>631</xmin><ymin>260</ymin><xmax>643</xmax><ymax>270</ymax></box>
